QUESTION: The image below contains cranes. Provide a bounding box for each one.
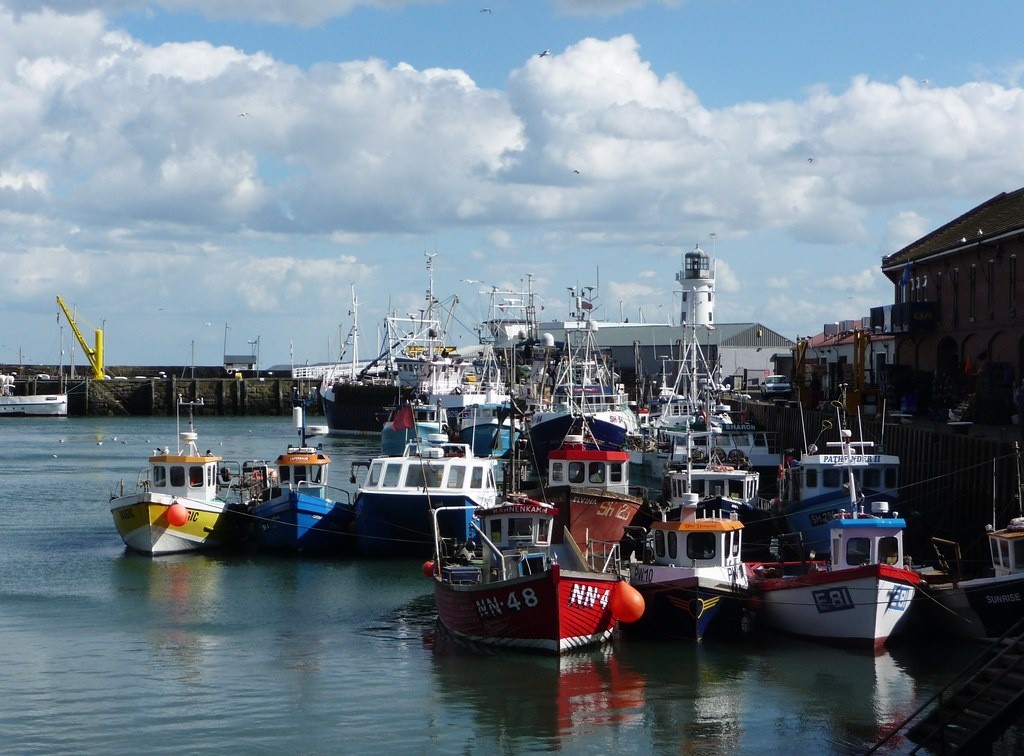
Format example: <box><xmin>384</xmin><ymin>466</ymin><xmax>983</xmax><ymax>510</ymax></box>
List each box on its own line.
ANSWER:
<box><xmin>56</xmin><ymin>296</ymin><xmax>106</xmax><ymax>380</ymax></box>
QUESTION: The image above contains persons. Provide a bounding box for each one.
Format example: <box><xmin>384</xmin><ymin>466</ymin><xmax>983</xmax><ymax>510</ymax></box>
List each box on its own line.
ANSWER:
<box><xmin>447</xmin><ymin>430</ymin><xmax>460</xmax><ymax>452</ymax></box>
<box><xmin>786</xmin><ymin>451</ymin><xmax>801</xmax><ymax>485</ymax></box>
<box><xmin>698</xmin><ymin>405</ymin><xmax>706</xmax><ymax>424</ymax></box>
<box><xmin>1014</xmin><ymin>379</ymin><xmax>1024</xmax><ymax>442</ymax></box>
<box><xmin>950</xmin><ymin>350</ymin><xmax>992</xmax><ymax>422</ymax></box>
<box><xmin>207</xmin><ymin>450</ymin><xmax>213</xmax><ymax>456</ymax></box>
<box><xmin>810</xmin><ymin>373</ymin><xmax>823</xmax><ymax>411</ymax></box>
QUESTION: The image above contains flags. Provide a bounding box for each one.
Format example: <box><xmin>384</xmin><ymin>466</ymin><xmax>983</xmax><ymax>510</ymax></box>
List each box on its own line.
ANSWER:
<box><xmin>581</xmin><ymin>302</ymin><xmax>592</xmax><ymax>309</ymax></box>
<box><xmin>390</xmin><ymin>404</ymin><xmax>414</xmax><ymax>431</ymax></box>
<box><xmin>898</xmin><ymin>261</ymin><xmax>909</xmax><ymax>287</ymax></box>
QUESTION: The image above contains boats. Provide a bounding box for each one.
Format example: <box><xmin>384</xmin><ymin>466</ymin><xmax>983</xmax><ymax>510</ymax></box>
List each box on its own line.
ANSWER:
<box><xmin>646</xmin><ymin>461</ymin><xmax>781</xmax><ymax>545</ymax></box>
<box><xmin>109</xmin><ymin>398</ymin><xmax>250</xmax><ymax>556</ymax></box>
<box><xmin>457</xmin><ymin>404</ymin><xmax>522</xmax><ymax>458</ymax></box>
<box><xmin>380</xmin><ymin>403</ymin><xmax>452</xmax><ymax>457</ymax></box>
<box><xmin>421</xmin><ymin>501</ymin><xmax>626</xmax><ymax>650</ymax></box>
<box><xmin>744</xmin><ymin>386</ymin><xmax>920</xmax><ymax>649</ymax></box>
<box><xmin>905</xmin><ymin>437</ymin><xmax>1024</xmax><ymax>648</ymax></box>
<box><xmin>522</xmin><ymin>405</ymin><xmax>644</xmax><ymax>455</ymax></box>
<box><xmin>507</xmin><ymin>436</ymin><xmax>646</xmax><ymax>559</ymax></box>
<box><xmin>245</xmin><ymin>446</ymin><xmax>352</xmax><ymax>553</ymax></box>
<box><xmin>746</xmin><ymin>647</ymin><xmax>917</xmax><ymax>754</ymax></box>
<box><xmin>624</xmin><ymin>515</ymin><xmax>746</xmax><ymax>643</ymax></box>
<box><xmin>322</xmin><ymin>368</ymin><xmax>739</xmax><ymax>436</ymax></box>
<box><xmin>646</xmin><ymin>419</ymin><xmax>780</xmax><ymax>484</ymax></box>
<box><xmin>432</xmin><ymin>651</ymin><xmax>647</xmax><ymax>756</ymax></box>
<box><xmin>0</xmin><ymin>386</ymin><xmax>70</xmax><ymax>419</ymax></box>
<box><xmin>741</xmin><ymin>559</ymin><xmax>781</xmax><ymax>636</ymax></box>
<box><xmin>351</xmin><ymin>439</ymin><xmax>499</xmax><ymax>550</ymax></box>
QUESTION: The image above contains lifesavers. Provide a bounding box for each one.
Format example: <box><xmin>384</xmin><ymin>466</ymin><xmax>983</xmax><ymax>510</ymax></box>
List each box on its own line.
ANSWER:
<box><xmin>518</xmin><ymin>496</ymin><xmax>553</xmax><ymax>509</ymax></box>
<box><xmin>713</xmin><ymin>466</ymin><xmax>735</xmax><ymax>471</ymax></box>
